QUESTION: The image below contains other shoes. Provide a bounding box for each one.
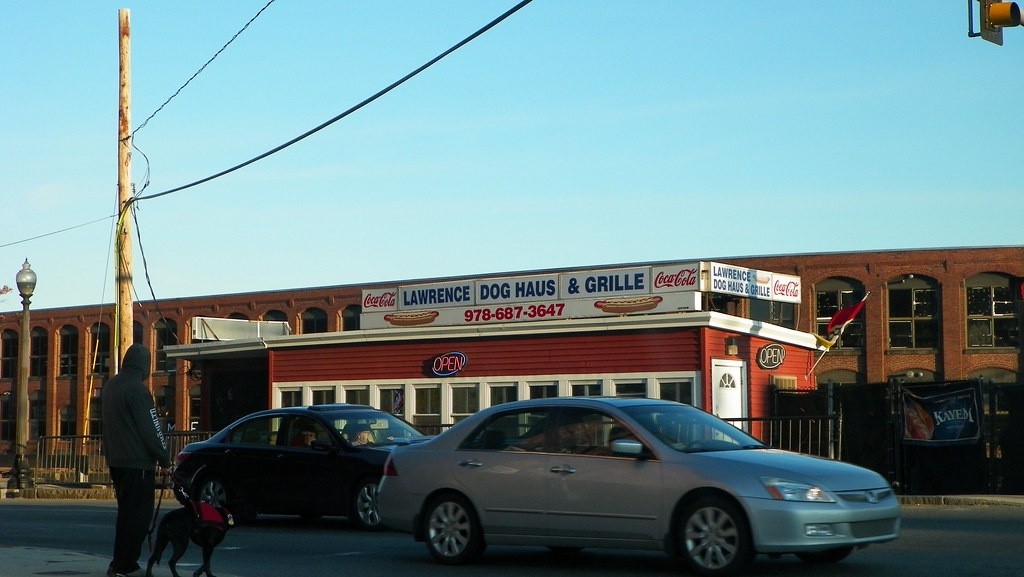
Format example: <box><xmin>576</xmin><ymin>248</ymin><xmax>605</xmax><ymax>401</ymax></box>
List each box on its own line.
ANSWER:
<box><xmin>106</xmin><ymin>566</ymin><xmax>148</xmax><ymax>577</ymax></box>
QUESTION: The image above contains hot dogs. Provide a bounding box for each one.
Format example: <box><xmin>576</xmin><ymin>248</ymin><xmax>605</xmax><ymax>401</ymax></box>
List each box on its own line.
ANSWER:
<box><xmin>753</xmin><ymin>275</ymin><xmax>770</xmax><ymax>284</ymax></box>
<box><xmin>903</xmin><ymin>394</ymin><xmax>935</xmax><ymax>441</ymax></box>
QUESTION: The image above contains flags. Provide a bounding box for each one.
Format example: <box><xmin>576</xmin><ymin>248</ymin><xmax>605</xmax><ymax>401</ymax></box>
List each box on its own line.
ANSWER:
<box><xmin>813</xmin><ymin>301</ymin><xmax>864</xmax><ymax>348</ymax></box>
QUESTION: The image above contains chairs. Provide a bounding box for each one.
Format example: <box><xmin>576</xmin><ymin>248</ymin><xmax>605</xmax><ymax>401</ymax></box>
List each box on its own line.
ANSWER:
<box><xmin>241</xmin><ymin>430</ymin><xmax>262</xmax><ymax>446</ymax></box>
<box><xmin>343</xmin><ymin>424</ymin><xmax>378</xmax><ymax>445</ymax></box>
<box><xmin>609</xmin><ymin>428</ymin><xmax>634</xmax><ymax>447</ymax></box>
<box><xmin>479</xmin><ymin>428</ymin><xmax>506</xmax><ymax>452</ymax></box>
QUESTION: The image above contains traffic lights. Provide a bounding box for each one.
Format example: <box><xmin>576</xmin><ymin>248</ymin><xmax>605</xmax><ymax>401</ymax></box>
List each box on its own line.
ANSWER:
<box><xmin>979</xmin><ymin>0</ymin><xmax>1021</xmax><ymax>46</ymax></box>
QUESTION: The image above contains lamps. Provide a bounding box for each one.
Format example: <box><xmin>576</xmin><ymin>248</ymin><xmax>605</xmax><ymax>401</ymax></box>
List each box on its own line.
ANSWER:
<box><xmin>728</xmin><ymin>337</ymin><xmax>739</xmax><ymax>356</ymax></box>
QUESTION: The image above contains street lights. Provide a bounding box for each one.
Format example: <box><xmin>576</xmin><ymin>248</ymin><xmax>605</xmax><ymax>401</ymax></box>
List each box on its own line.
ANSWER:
<box><xmin>7</xmin><ymin>258</ymin><xmax>40</xmax><ymax>491</ymax></box>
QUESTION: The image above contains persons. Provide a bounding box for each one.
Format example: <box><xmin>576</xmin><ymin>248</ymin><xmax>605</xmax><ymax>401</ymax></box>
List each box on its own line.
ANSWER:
<box><xmin>102</xmin><ymin>344</ymin><xmax>171</xmax><ymax>577</ymax></box>
<box><xmin>299</xmin><ymin>425</ymin><xmax>317</xmax><ymax>446</ymax></box>
<box><xmin>354</xmin><ymin>424</ymin><xmax>374</xmax><ymax>445</ymax></box>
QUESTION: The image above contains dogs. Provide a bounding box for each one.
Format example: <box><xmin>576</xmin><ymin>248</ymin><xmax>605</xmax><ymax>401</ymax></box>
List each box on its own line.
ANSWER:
<box><xmin>146</xmin><ymin>500</ymin><xmax>245</xmax><ymax>577</ymax></box>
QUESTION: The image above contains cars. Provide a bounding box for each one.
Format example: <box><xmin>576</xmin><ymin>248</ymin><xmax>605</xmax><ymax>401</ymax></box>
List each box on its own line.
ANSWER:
<box><xmin>379</xmin><ymin>396</ymin><xmax>905</xmax><ymax>577</ymax></box>
<box><xmin>165</xmin><ymin>402</ymin><xmax>422</xmax><ymax>536</ymax></box>
